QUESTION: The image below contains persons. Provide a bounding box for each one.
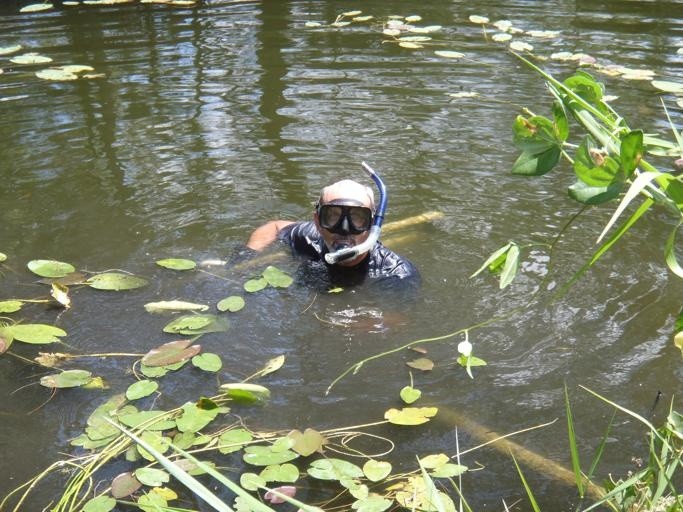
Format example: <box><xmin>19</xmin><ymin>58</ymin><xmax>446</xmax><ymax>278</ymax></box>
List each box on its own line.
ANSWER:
<box><xmin>226</xmin><ymin>176</ymin><xmax>422</xmax><ymax>327</ymax></box>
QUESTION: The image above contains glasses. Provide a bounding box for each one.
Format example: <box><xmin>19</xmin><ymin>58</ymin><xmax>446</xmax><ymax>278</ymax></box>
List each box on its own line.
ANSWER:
<box><xmin>314</xmin><ymin>200</ymin><xmax>374</xmax><ymax>236</ymax></box>
